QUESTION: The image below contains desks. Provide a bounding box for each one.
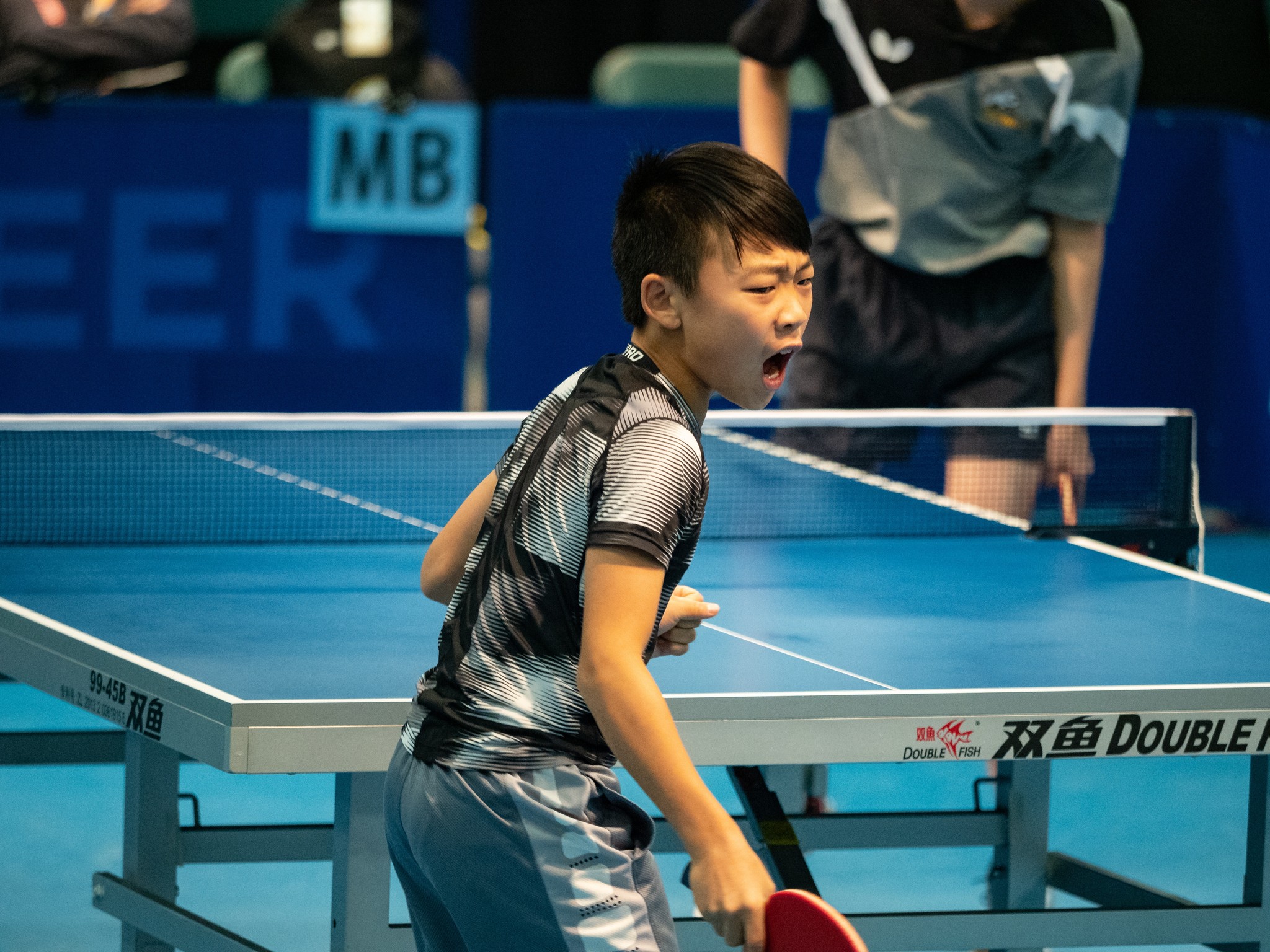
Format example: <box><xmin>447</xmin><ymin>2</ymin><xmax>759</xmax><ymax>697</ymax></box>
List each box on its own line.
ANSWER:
<box><xmin>0</xmin><ymin>402</ymin><xmax>1270</xmax><ymax>952</ymax></box>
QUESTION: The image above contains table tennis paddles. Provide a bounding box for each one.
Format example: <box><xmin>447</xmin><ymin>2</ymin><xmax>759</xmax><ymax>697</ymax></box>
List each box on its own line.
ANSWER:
<box><xmin>1058</xmin><ymin>470</ymin><xmax>1075</xmax><ymax>525</ymax></box>
<box><xmin>680</xmin><ymin>861</ymin><xmax>871</xmax><ymax>952</ymax></box>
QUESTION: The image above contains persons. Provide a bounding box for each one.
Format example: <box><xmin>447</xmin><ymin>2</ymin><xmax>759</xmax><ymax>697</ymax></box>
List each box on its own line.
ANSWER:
<box><xmin>0</xmin><ymin>0</ymin><xmax>198</xmax><ymax>99</ymax></box>
<box><xmin>728</xmin><ymin>0</ymin><xmax>1144</xmax><ymax>528</ymax></box>
<box><xmin>387</xmin><ymin>140</ymin><xmax>815</xmax><ymax>952</ymax></box>
